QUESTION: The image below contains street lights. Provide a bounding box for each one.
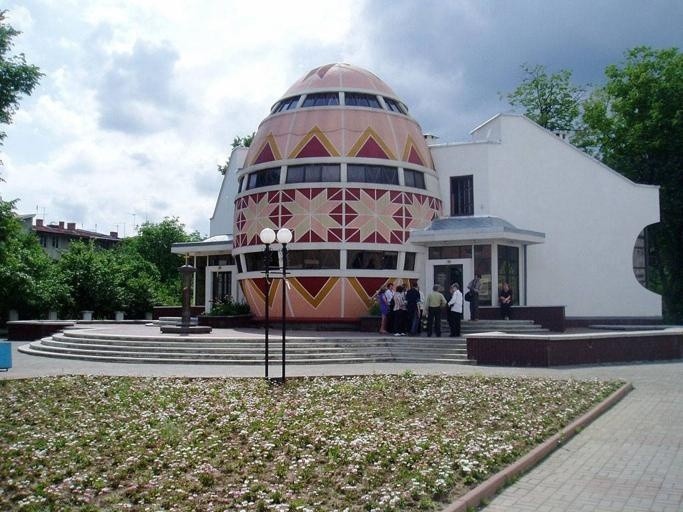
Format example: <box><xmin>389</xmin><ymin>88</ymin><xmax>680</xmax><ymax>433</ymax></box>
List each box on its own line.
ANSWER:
<box><xmin>276</xmin><ymin>228</ymin><xmax>292</xmax><ymax>384</ymax></box>
<box><xmin>259</xmin><ymin>228</ymin><xmax>275</xmax><ymax>378</ymax></box>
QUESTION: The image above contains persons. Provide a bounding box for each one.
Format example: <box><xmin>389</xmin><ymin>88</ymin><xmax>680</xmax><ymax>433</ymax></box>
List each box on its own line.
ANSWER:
<box><xmin>467</xmin><ymin>273</ymin><xmax>482</xmax><ymax>320</ymax></box>
<box><xmin>379</xmin><ymin>282</ymin><xmax>462</xmax><ymax>337</ymax></box>
<box><xmin>500</xmin><ymin>283</ymin><xmax>513</xmax><ymax>320</ymax></box>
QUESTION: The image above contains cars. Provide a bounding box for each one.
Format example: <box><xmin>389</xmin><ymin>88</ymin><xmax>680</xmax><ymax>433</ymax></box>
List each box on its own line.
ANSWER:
<box><xmin>476</xmin><ymin>274</ymin><xmax>505</xmax><ymax>302</ymax></box>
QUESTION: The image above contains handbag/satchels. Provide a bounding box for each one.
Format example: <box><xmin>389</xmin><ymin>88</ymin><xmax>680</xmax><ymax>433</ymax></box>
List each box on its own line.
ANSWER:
<box><xmin>390</xmin><ymin>298</ymin><xmax>394</xmax><ymax>309</ymax></box>
<box><xmin>465</xmin><ymin>292</ymin><xmax>474</xmax><ymax>301</ymax></box>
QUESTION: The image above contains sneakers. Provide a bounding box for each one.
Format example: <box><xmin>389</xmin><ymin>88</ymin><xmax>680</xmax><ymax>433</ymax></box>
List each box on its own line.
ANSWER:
<box><xmin>393</xmin><ymin>333</ymin><xmax>407</xmax><ymax>336</ymax></box>
